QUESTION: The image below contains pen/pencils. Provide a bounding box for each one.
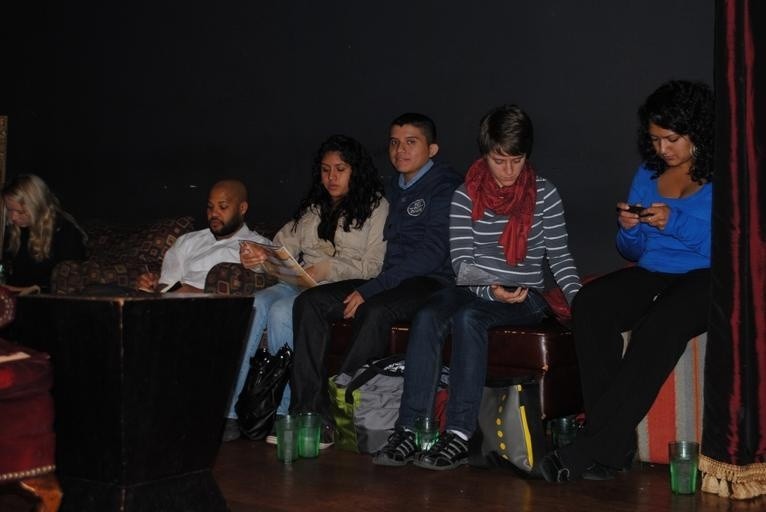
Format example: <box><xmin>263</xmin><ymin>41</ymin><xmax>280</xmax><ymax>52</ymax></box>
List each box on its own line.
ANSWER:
<box><xmin>145</xmin><ymin>263</ymin><xmax>154</xmax><ymax>289</ymax></box>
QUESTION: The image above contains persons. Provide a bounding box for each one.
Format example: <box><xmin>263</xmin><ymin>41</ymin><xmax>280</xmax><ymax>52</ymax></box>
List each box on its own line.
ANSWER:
<box><xmin>136</xmin><ymin>178</ymin><xmax>269</xmax><ymax>296</ymax></box>
<box><xmin>537</xmin><ymin>75</ymin><xmax>714</xmax><ymax>486</ymax></box>
<box><xmin>221</xmin><ymin>129</ymin><xmax>389</xmax><ymax>447</ymax></box>
<box><xmin>0</xmin><ymin>174</ymin><xmax>93</xmax><ymax>297</ymax></box>
<box><xmin>370</xmin><ymin>99</ymin><xmax>583</xmax><ymax>472</ymax></box>
<box><xmin>264</xmin><ymin>109</ymin><xmax>459</xmax><ymax>451</ymax></box>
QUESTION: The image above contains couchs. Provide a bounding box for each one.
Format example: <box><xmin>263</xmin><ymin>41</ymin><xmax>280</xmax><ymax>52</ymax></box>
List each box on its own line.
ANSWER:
<box><xmin>0</xmin><ymin>286</ymin><xmax>68</xmax><ymax>512</ymax></box>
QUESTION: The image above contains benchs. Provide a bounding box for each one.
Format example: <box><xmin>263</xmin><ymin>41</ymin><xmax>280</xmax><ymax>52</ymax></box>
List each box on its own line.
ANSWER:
<box><xmin>325</xmin><ymin>319</ymin><xmax>578</xmax><ymax>421</ymax></box>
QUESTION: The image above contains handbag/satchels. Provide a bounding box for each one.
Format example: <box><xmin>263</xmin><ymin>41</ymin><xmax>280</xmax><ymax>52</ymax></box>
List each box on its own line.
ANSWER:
<box><xmin>233</xmin><ymin>343</ymin><xmax>294</xmax><ymax>442</ymax></box>
<box><xmin>328</xmin><ymin>357</ymin><xmax>452</xmax><ymax>455</ymax></box>
<box><xmin>478</xmin><ymin>386</ymin><xmax>535</xmax><ymax>472</ymax></box>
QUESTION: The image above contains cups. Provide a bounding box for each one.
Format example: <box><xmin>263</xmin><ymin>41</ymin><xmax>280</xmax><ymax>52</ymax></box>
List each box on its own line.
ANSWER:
<box><xmin>295</xmin><ymin>410</ymin><xmax>322</xmax><ymax>460</ymax></box>
<box><xmin>414</xmin><ymin>415</ymin><xmax>441</xmax><ymax>452</ymax></box>
<box><xmin>272</xmin><ymin>413</ymin><xmax>302</xmax><ymax>464</ymax></box>
<box><xmin>667</xmin><ymin>439</ymin><xmax>703</xmax><ymax>494</ymax></box>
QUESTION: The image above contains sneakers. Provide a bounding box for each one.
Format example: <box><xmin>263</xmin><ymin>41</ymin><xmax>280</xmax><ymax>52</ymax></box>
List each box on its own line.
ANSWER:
<box><xmin>223</xmin><ymin>414</ymin><xmax>288</xmax><ymax>443</ymax></box>
<box><xmin>371</xmin><ymin>429</ymin><xmax>483</xmax><ymax>471</ymax></box>
<box><xmin>539</xmin><ymin>448</ymin><xmax>636</xmax><ymax>484</ymax></box>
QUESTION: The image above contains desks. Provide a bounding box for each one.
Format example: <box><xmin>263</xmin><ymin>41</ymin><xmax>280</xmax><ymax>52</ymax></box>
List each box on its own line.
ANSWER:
<box><xmin>3</xmin><ymin>291</ymin><xmax>254</xmax><ymax>512</ymax></box>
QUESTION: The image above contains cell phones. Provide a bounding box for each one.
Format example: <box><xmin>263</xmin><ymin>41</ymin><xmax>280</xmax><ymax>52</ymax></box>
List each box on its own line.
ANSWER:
<box><xmin>625</xmin><ymin>206</ymin><xmax>654</xmax><ymax>217</ymax></box>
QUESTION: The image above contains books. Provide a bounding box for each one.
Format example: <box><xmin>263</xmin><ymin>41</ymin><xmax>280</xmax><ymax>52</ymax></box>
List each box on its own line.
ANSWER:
<box><xmin>137</xmin><ymin>279</ymin><xmax>184</xmax><ymax>295</ymax></box>
<box><xmin>236</xmin><ymin>238</ymin><xmax>319</xmax><ymax>289</ymax></box>
